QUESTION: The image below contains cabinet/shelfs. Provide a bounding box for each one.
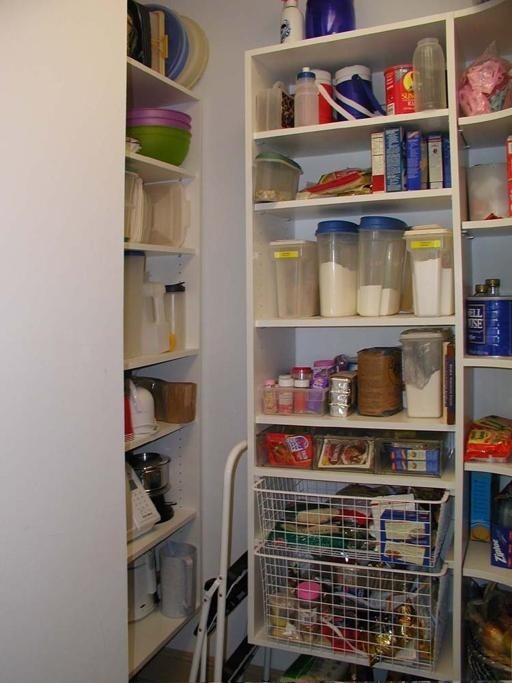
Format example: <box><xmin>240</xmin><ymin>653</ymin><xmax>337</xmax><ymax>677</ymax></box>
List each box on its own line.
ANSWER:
<box><xmin>243</xmin><ymin>1</ymin><xmax>512</xmax><ymax>683</ymax></box>
<box><xmin>124</xmin><ymin>56</ymin><xmax>203</xmax><ymax>680</ymax></box>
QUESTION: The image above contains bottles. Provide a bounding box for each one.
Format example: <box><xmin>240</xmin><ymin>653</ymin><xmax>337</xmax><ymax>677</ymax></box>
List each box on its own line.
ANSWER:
<box><xmin>260</xmin><ymin>367</ymin><xmax>314</xmax><ymax>413</ymax></box>
<box><xmin>413</xmin><ymin>38</ymin><xmax>445</xmax><ymax>112</ymax></box>
<box><xmin>163</xmin><ymin>282</ymin><xmax>186</xmax><ymax>353</ymax></box>
<box><xmin>270</xmin><ymin>579</ymin><xmax>320</xmax><ymax>643</ymax></box>
<box><xmin>473</xmin><ymin>277</ymin><xmax>501</xmax><ymax>297</ymax></box>
<box><xmin>294</xmin><ymin>72</ymin><xmax>320</xmax><ymax>128</ymax></box>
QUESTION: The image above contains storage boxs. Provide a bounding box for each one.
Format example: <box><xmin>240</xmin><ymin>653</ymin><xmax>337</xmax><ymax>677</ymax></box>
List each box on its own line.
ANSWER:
<box><xmin>398</xmin><ymin>329</ymin><xmax>455</xmax><ymax>417</ymax></box>
<box><xmin>144</xmin><ymin>183</ymin><xmax>191</xmax><ymax>247</ymax></box>
<box><xmin>125</xmin><ymin>171</ymin><xmax>154</xmax><ymax>244</ymax></box>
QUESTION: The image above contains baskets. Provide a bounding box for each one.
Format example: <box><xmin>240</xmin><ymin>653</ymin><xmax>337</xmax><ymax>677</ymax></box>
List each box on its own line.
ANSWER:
<box><xmin>250</xmin><ymin>477</ymin><xmax>450</xmax><ymax>676</ymax></box>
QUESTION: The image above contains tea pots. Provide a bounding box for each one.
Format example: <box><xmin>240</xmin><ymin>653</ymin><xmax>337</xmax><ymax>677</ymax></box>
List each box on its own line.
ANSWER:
<box><xmin>159</xmin><ymin>539</ymin><xmax>197</xmax><ymax>618</ymax></box>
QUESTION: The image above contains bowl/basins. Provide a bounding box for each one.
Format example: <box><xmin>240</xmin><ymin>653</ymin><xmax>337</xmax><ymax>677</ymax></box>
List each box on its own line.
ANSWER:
<box><xmin>126</xmin><ymin>107</ymin><xmax>193</xmax><ymax>166</ymax></box>
